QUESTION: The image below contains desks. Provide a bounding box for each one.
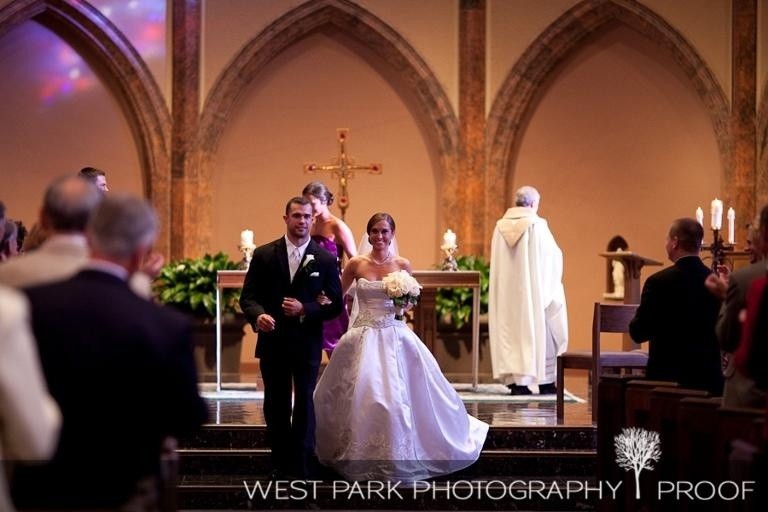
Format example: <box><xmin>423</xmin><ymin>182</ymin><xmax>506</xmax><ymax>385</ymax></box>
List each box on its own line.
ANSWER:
<box><xmin>216</xmin><ymin>269</ymin><xmax>481</xmax><ymax>392</ymax></box>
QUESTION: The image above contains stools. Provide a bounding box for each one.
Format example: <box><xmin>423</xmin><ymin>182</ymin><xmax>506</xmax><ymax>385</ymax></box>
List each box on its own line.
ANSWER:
<box><xmin>620</xmin><ymin>380</ymin><xmax>678</xmax><ymax>436</ymax></box>
<box><xmin>645</xmin><ymin>386</ymin><xmax>709</xmax><ymax>467</ymax></box>
<box><xmin>712</xmin><ymin>405</ymin><xmax>768</xmax><ymax>492</ymax></box>
<box><xmin>672</xmin><ymin>396</ymin><xmax>722</xmax><ymax>476</ymax></box>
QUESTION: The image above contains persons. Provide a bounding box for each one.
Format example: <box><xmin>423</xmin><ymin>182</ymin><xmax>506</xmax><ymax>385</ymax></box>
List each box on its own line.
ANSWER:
<box><xmin>487</xmin><ymin>186</ymin><xmax>570</xmax><ymax>394</ymax></box>
<box><xmin>314</xmin><ymin>213</ymin><xmax>489</xmax><ymax>489</ymax></box>
<box><xmin>302</xmin><ymin>181</ymin><xmax>356</xmax><ymax>360</ymax></box>
<box><xmin>628</xmin><ymin>205</ymin><xmax>767</xmax><ymax>408</ymax></box>
<box><xmin>1</xmin><ymin>167</ymin><xmax>209</xmax><ymax>512</ymax></box>
<box><xmin>239</xmin><ymin>197</ymin><xmax>343</xmax><ymax>480</ymax></box>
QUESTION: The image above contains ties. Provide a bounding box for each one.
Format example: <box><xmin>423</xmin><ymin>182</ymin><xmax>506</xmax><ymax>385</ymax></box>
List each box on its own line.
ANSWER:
<box><xmin>287</xmin><ymin>247</ymin><xmax>302</xmax><ymax>281</ymax></box>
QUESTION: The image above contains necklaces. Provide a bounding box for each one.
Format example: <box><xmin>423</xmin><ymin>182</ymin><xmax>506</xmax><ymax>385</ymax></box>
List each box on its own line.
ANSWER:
<box><xmin>373</xmin><ymin>251</ymin><xmax>391</xmax><ymax>269</ymax></box>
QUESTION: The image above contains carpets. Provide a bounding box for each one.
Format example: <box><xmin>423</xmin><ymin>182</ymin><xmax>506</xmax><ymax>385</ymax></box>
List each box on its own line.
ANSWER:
<box><xmin>198</xmin><ymin>382</ymin><xmax>583</xmax><ymax>401</ymax></box>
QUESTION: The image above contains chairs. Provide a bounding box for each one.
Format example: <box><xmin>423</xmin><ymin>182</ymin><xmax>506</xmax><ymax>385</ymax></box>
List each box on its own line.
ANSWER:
<box><xmin>557</xmin><ymin>302</ymin><xmax>650</xmax><ymax>424</ymax></box>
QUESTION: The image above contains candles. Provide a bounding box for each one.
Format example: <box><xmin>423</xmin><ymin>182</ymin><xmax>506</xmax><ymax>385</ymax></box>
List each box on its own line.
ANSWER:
<box><xmin>444</xmin><ymin>229</ymin><xmax>456</xmax><ymax>246</ymax></box>
<box><xmin>241</xmin><ymin>228</ymin><xmax>253</xmax><ymax>247</ymax></box>
<box><xmin>727</xmin><ymin>207</ymin><xmax>736</xmax><ymax>243</ymax></box>
<box><xmin>711</xmin><ymin>198</ymin><xmax>722</xmax><ymax>229</ymax></box>
<box><xmin>696</xmin><ymin>206</ymin><xmax>703</xmax><ymax>244</ymax></box>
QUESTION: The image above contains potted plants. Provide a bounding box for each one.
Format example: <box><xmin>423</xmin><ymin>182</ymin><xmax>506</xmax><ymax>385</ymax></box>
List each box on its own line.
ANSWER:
<box><xmin>149</xmin><ymin>250</ymin><xmax>249</xmax><ymax>346</ymax></box>
<box><xmin>437</xmin><ymin>254</ymin><xmax>489</xmax><ymax>339</ymax></box>
